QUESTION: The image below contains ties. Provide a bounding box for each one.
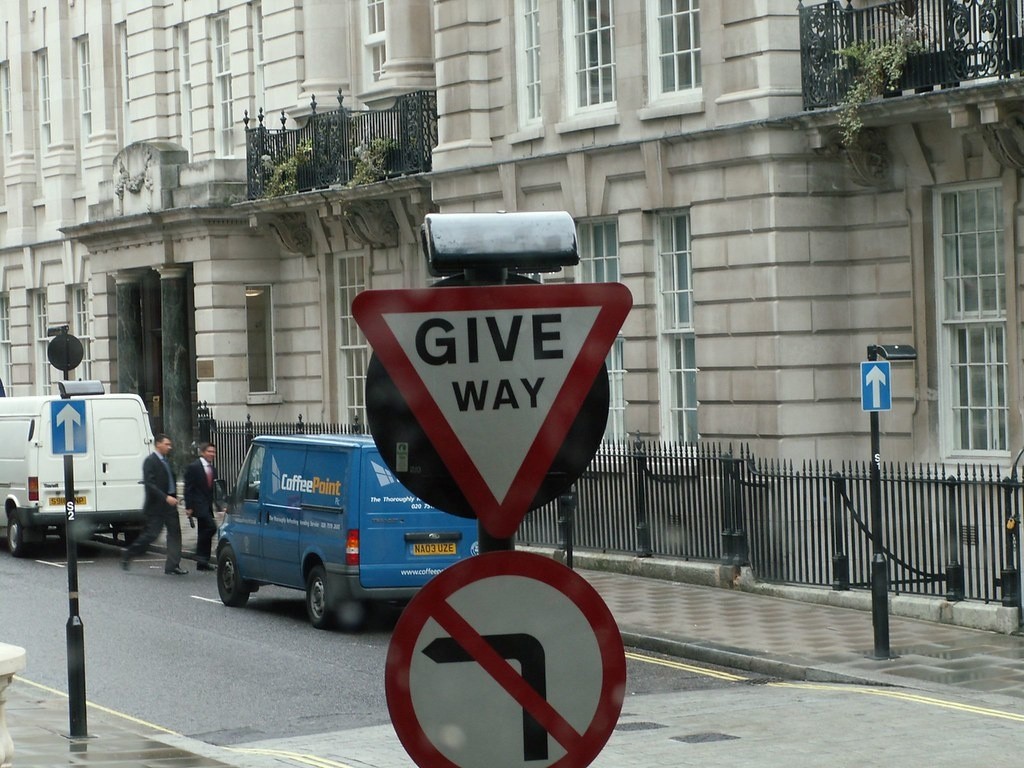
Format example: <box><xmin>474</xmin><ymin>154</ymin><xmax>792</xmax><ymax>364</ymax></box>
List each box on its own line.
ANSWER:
<box><xmin>207</xmin><ymin>464</ymin><xmax>212</xmax><ymax>487</ymax></box>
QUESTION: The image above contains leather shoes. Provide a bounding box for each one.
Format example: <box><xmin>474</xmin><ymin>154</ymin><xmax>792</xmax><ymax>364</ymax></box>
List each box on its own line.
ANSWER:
<box><xmin>197</xmin><ymin>562</ymin><xmax>214</xmax><ymax>571</ymax></box>
<box><xmin>121</xmin><ymin>555</ymin><xmax>130</xmax><ymax>570</ymax></box>
<box><xmin>165</xmin><ymin>565</ymin><xmax>189</xmax><ymax>575</ymax></box>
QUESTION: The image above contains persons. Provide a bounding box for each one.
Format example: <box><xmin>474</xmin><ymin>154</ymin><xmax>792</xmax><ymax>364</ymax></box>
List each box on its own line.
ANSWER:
<box><xmin>182</xmin><ymin>441</ymin><xmax>219</xmax><ymax>572</ymax></box>
<box><xmin>119</xmin><ymin>433</ymin><xmax>189</xmax><ymax>575</ymax></box>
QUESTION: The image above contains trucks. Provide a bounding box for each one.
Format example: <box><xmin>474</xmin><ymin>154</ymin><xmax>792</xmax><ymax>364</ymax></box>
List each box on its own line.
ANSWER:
<box><xmin>0</xmin><ymin>393</ymin><xmax>157</xmax><ymax>558</ymax></box>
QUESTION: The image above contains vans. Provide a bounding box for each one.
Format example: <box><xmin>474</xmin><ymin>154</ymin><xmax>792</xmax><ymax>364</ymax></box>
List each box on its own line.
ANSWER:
<box><xmin>213</xmin><ymin>435</ymin><xmax>479</xmax><ymax>631</ymax></box>
